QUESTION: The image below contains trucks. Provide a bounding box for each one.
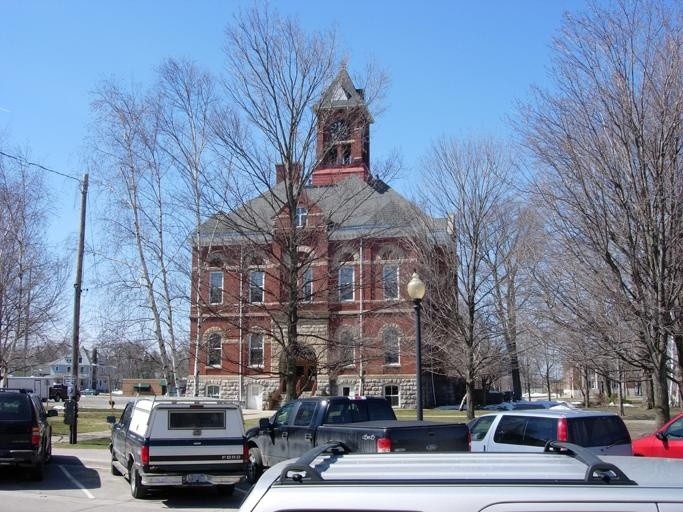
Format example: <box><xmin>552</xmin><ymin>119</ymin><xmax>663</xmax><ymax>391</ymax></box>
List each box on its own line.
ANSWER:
<box><xmin>0</xmin><ymin>376</ymin><xmax>81</xmax><ymax>402</ymax></box>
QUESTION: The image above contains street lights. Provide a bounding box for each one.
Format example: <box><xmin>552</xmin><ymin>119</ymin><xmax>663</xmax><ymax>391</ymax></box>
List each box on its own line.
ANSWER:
<box><xmin>407</xmin><ymin>267</ymin><xmax>426</xmax><ymax>421</ymax></box>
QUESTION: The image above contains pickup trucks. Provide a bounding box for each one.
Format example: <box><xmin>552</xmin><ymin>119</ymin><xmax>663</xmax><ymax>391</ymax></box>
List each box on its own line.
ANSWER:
<box><xmin>244</xmin><ymin>394</ymin><xmax>472</xmax><ymax>483</ymax></box>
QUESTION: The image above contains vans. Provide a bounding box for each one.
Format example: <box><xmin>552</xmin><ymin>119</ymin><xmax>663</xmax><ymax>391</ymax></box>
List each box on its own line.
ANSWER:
<box><xmin>465</xmin><ymin>409</ymin><xmax>634</xmax><ymax>457</ymax></box>
<box><xmin>105</xmin><ymin>395</ymin><xmax>250</xmax><ymax>498</ymax></box>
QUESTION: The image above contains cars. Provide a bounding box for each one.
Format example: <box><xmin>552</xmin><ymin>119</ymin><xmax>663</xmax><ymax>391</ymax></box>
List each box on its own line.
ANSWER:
<box><xmin>235</xmin><ymin>440</ymin><xmax>683</xmax><ymax>512</ymax></box>
<box><xmin>630</xmin><ymin>411</ymin><xmax>683</xmax><ymax>458</ymax></box>
<box><xmin>80</xmin><ymin>389</ymin><xmax>96</xmax><ymax>395</ymax></box>
<box><xmin>0</xmin><ymin>387</ymin><xmax>58</xmax><ymax>482</ymax></box>
